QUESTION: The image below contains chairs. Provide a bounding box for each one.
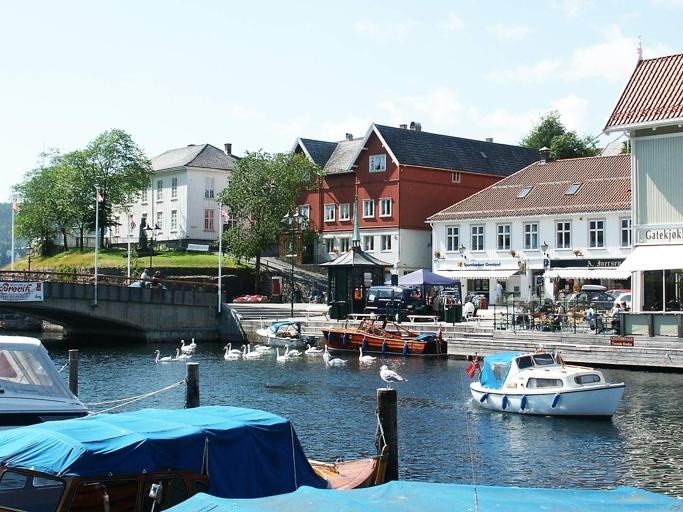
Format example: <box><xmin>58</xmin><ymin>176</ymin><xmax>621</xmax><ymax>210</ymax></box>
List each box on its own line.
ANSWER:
<box><xmin>584</xmin><ymin>315</ymin><xmax>597</xmax><ymax>335</ymax></box>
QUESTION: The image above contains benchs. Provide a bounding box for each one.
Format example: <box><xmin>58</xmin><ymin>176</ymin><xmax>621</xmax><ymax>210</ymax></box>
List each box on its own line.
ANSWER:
<box><xmin>186</xmin><ymin>244</ymin><xmax>209</xmax><ymax>255</ymax></box>
<box><xmin>407</xmin><ymin>315</ymin><xmax>441</xmax><ymax>322</ymax></box>
<box><xmin>347</xmin><ymin>314</ymin><xmax>382</xmax><ymax>320</ymax></box>
<box><xmin>610</xmin><ymin>336</ymin><xmax>634</xmax><ymax>347</ymax></box>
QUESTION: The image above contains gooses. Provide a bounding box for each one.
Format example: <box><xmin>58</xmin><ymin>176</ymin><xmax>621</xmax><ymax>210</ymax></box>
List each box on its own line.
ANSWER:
<box><xmin>153</xmin><ymin>337</ymin><xmax>197</xmax><ymax>363</ymax></box>
<box><xmin>283</xmin><ymin>344</ymin><xmax>303</xmax><ymax>357</ymax></box>
<box><xmin>358</xmin><ymin>345</ymin><xmax>378</xmax><ymax>362</ymax></box>
<box><xmin>325</xmin><ymin>352</ymin><xmax>347</xmax><ymax>366</ymax></box>
<box><xmin>304</xmin><ymin>344</ymin><xmax>324</xmax><ymax>353</ymax></box>
<box><xmin>275</xmin><ymin>347</ymin><xmax>289</xmax><ymax>363</ymax></box>
<box><xmin>322</xmin><ymin>343</ymin><xmax>331</xmax><ymax>363</ymax></box>
<box><xmin>223</xmin><ymin>342</ymin><xmax>272</xmax><ymax>360</ymax></box>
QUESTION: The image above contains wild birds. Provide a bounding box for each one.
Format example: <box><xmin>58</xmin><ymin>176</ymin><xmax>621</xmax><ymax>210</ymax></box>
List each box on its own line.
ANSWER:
<box><xmin>379</xmin><ymin>365</ymin><xmax>408</xmax><ymax>389</ymax></box>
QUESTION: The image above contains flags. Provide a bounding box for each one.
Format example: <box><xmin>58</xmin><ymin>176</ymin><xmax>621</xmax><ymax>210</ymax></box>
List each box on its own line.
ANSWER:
<box><xmin>555</xmin><ymin>350</ymin><xmax>565</xmax><ymax>369</ymax></box>
<box><xmin>465</xmin><ymin>358</ymin><xmax>479</xmax><ymax>379</ymax></box>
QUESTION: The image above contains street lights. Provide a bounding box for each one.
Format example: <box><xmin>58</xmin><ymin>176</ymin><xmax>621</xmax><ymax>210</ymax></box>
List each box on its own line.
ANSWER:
<box><xmin>143</xmin><ymin>224</ymin><xmax>161</xmax><ymax>268</ymax></box>
<box><xmin>25</xmin><ymin>245</ymin><xmax>35</xmax><ymax>281</ymax></box>
<box><xmin>283</xmin><ymin>209</ymin><xmax>304</xmax><ymax>318</ymax></box>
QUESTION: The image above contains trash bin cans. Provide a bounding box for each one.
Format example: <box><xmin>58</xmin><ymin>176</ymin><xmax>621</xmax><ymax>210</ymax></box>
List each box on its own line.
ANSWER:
<box><xmin>445</xmin><ymin>304</ymin><xmax>462</xmax><ymax>323</ymax></box>
<box><xmin>329</xmin><ymin>300</ymin><xmax>346</xmax><ymax>322</ymax></box>
<box><xmin>293</xmin><ymin>290</ymin><xmax>303</xmax><ymax>303</ymax></box>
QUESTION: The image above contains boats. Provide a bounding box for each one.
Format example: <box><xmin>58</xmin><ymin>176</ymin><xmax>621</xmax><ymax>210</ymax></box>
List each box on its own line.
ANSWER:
<box><xmin>0</xmin><ymin>313</ymin><xmax>43</xmax><ymax>331</ymax></box>
<box><xmin>256</xmin><ymin>321</ymin><xmax>447</xmax><ymax>354</ymax></box>
<box><xmin>470</xmin><ymin>352</ymin><xmax>626</xmax><ymax>419</ymax></box>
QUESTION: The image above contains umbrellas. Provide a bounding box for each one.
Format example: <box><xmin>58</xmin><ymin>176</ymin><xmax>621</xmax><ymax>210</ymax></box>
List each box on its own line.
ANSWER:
<box><xmin>383</xmin><ymin>267</ymin><xmax>459</xmax><ymax>305</ymax></box>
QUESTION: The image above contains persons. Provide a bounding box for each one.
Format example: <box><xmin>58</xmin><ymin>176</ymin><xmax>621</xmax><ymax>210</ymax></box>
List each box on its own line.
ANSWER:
<box><xmin>550</xmin><ymin>301</ymin><xmax>627</xmax><ymax>334</ymax></box>
<box><xmin>150</xmin><ymin>270</ymin><xmax>161</xmax><ymax>289</ymax></box>
<box><xmin>140</xmin><ymin>268</ymin><xmax>150</xmax><ymax>288</ymax></box>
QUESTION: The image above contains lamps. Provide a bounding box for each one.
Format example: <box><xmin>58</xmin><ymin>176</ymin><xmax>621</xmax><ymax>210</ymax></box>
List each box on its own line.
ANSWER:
<box><xmin>459</xmin><ymin>245</ymin><xmax>466</xmax><ymax>259</ymax></box>
<box><xmin>540</xmin><ymin>241</ymin><xmax>549</xmax><ymax>257</ymax></box>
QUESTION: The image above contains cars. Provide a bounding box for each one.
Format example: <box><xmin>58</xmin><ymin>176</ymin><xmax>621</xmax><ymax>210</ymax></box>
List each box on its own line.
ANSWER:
<box><xmin>364</xmin><ymin>286</ymin><xmax>414</xmax><ymax>321</ymax></box>
<box><xmin>567</xmin><ymin>289</ymin><xmax>631</xmax><ymax>312</ymax></box>
